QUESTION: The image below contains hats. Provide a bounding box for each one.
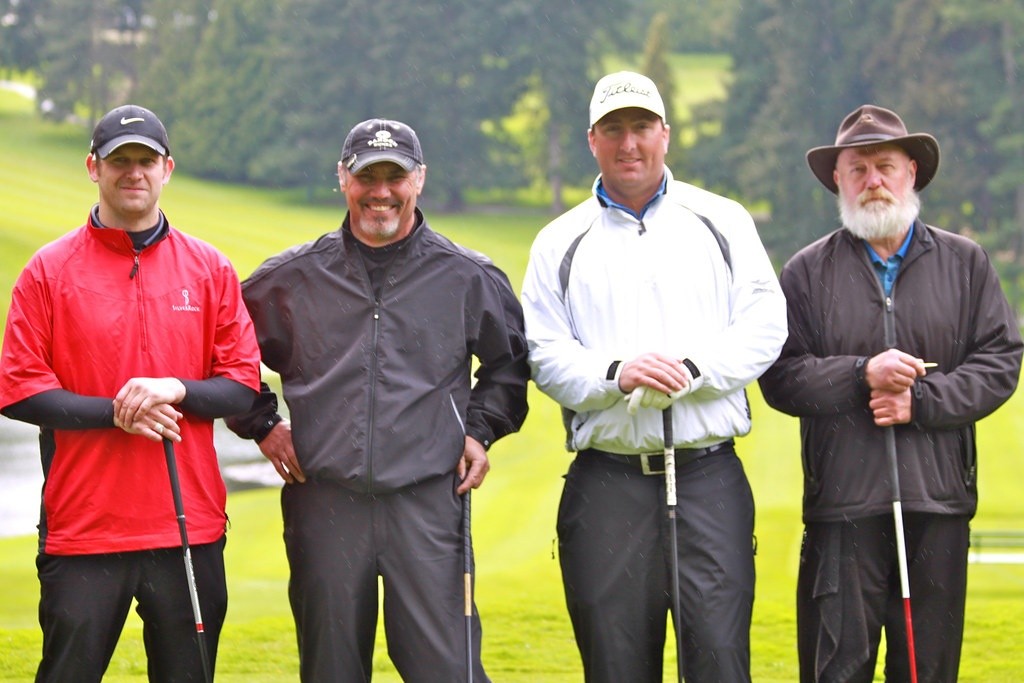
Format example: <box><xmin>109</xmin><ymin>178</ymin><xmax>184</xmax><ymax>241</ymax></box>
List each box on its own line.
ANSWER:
<box><xmin>91</xmin><ymin>105</ymin><xmax>171</xmax><ymax>160</ymax></box>
<box><xmin>589</xmin><ymin>71</ymin><xmax>666</xmax><ymax>128</ymax></box>
<box><xmin>341</xmin><ymin>118</ymin><xmax>423</xmax><ymax>177</ymax></box>
<box><xmin>806</xmin><ymin>104</ymin><xmax>941</xmax><ymax>197</ymax></box>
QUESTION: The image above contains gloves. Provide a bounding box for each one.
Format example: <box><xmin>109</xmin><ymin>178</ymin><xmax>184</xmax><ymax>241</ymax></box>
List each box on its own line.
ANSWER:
<box><xmin>623</xmin><ymin>380</ymin><xmax>690</xmax><ymax>415</ymax></box>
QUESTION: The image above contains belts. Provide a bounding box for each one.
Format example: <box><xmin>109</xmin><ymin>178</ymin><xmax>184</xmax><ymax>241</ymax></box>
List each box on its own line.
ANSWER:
<box><xmin>577</xmin><ymin>437</ymin><xmax>735</xmax><ymax>475</ymax></box>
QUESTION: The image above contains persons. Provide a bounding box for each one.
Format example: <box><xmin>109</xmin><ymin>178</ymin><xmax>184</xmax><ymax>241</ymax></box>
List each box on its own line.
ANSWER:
<box><xmin>0</xmin><ymin>105</ymin><xmax>264</xmax><ymax>683</ymax></box>
<box><xmin>243</xmin><ymin>115</ymin><xmax>529</xmax><ymax>683</ymax></box>
<box><xmin>758</xmin><ymin>104</ymin><xmax>1024</xmax><ymax>682</ymax></box>
<box><xmin>520</xmin><ymin>69</ymin><xmax>792</xmax><ymax>682</ymax></box>
